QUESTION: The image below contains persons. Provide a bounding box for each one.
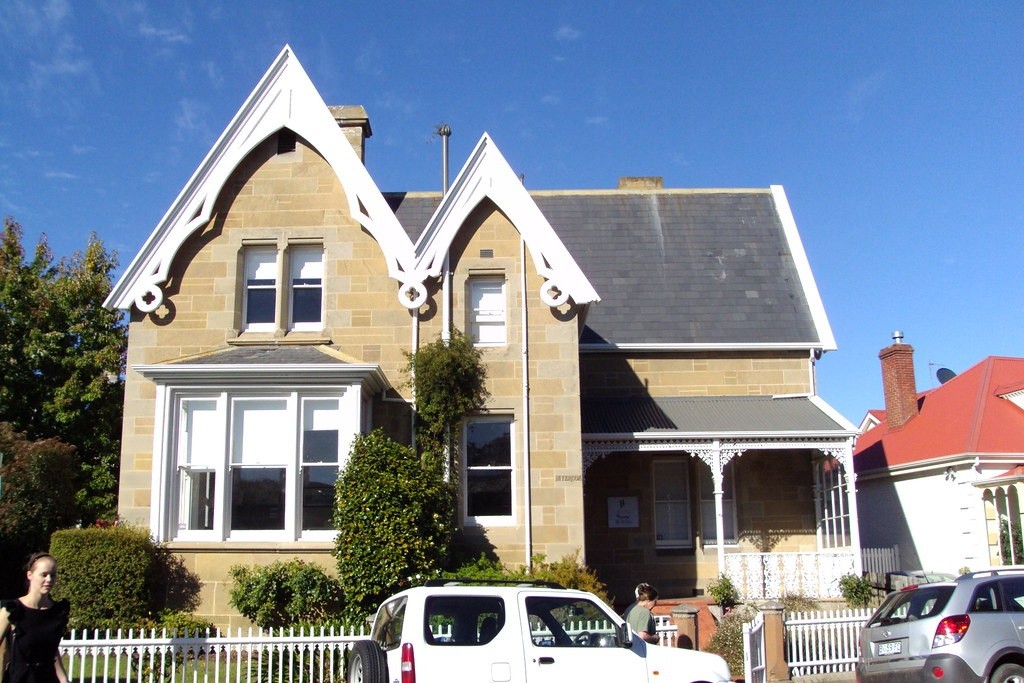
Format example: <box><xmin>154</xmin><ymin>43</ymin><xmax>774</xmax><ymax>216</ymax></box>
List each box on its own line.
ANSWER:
<box><xmin>0</xmin><ymin>552</ymin><xmax>70</xmax><ymax>683</ymax></box>
<box><xmin>623</xmin><ymin>583</ymin><xmax>660</xmax><ymax>645</ymax></box>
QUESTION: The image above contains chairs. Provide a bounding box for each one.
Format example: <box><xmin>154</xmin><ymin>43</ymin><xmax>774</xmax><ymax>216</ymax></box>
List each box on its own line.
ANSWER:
<box><xmin>979</xmin><ymin>600</ymin><xmax>993</xmax><ymax>610</ymax></box>
<box><xmin>478</xmin><ymin>617</ymin><xmax>497</xmax><ymax>643</ymax></box>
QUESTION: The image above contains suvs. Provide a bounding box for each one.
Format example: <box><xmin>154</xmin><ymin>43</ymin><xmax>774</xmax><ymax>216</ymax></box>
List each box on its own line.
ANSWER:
<box><xmin>348</xmin><ymin>579</ymin><xmax>735</xmax><ymax>683</ymax></box>
<box><xmin>856</xmin><ymin>566</ymin><xmax>1024</xmax><ymax>683</ymax></box>
